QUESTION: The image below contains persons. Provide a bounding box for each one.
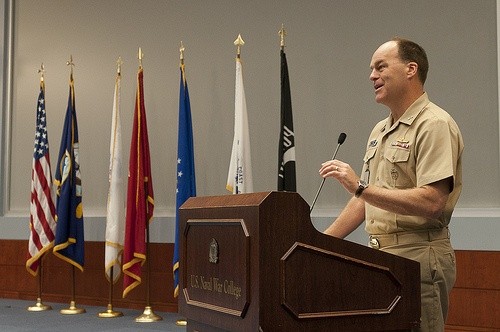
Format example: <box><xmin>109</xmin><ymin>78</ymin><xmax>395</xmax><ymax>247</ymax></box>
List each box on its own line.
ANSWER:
<box><xmin>318</xmin><ymin>36</ymin><xmax>464</xmax><ymax>332</ymax></box>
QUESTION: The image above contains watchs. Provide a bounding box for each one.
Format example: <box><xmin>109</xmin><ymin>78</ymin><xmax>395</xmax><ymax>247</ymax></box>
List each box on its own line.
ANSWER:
<box><xmin>354</xmin><ymin>181</ymin><xmax>369</xmax><ymax>199</ymax></box>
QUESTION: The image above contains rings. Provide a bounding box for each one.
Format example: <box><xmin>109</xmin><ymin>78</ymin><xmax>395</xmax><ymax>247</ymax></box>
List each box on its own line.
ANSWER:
<box><xmin>335</xmin><ymin>166</ymin><xmax>340</xmax><ymax>171</ymax></box>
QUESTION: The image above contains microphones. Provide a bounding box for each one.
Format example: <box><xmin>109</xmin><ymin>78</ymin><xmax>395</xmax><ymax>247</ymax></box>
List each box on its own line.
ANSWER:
<box><xmin>309</xmin><ymin>132</ymin><xmax>347</xmax><ymax>212</ymax></box>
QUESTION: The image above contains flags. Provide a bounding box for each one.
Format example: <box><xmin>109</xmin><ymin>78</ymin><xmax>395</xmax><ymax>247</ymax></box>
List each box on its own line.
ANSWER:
<box><xmin>53</xmin><ymin>86</ymin><xmax>85</xmax><ymax>271</ymax></box>
<box><xmin>225</xmin><ymin>57</ymin><xmax>254</xmax><ymax>194</ymax></box>
<box><xmin>103</xmin><ymin>78</ymin><xmax>128</xmax><ymax>282</ymax></box>
<box><xmin>122</xmin><ymin>66</ymin><xmax>154</xmax><ymax>300</ymax></box>
<box><xmin>170</xmin><ymin>69</ymin><xmax>197</xmax><ymax>297</ymax></box>
<box><xmin>25</xmin><ymin>89</ymin><xmax>58</xmax><ymax>276</ymax></box>
<box><xmin>275</xmin><ymin>51</ymin><xmax>297</xmax><ymax>192</ymax></box>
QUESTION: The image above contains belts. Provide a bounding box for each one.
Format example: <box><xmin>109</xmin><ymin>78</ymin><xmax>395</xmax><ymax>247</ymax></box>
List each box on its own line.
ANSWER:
<box><xmin>369</xmin><ymin>228</ymin><xmax>450</xmax><ymax>250</ymax></box>
